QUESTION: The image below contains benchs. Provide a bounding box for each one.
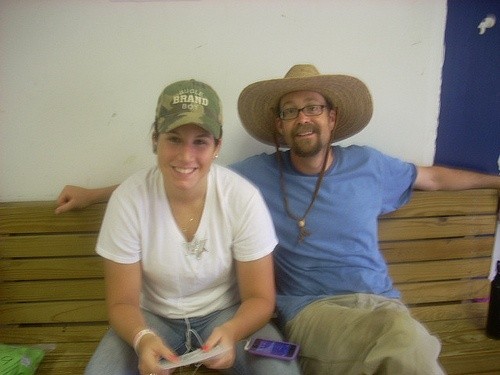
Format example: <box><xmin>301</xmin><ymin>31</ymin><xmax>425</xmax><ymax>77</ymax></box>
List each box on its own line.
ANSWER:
<box><xmin>0</xmin><ymin>188</ymin><xmax>500</xmax><ymax>375</ymax></box>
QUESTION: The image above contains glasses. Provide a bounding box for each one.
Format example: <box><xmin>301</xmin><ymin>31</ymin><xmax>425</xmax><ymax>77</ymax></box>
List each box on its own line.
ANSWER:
<box><xmin>280</xmin><ymin>105</ymin><xmax>333</xmax><ymax>120</ymax></box>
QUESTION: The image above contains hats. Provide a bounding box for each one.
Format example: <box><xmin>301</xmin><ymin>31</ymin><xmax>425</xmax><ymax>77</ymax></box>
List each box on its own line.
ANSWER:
<box><xmin>155</xmin><ymin>79</ymin><xmax>223</xmax><ymax>140</ymax></box>
<box><xmin>238</xmin><ymin>65</ymin><xmax>374</xmax><ymax>148</ymax></box>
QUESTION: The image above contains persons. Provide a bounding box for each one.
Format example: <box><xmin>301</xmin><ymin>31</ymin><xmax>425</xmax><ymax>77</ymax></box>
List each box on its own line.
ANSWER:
<box><xmin>84</xmin><ymin>80</ymin><xmax>300</xmax><ymax>375</ymax></box>
<box><xmin>55</xmin><ymin>64</ymin><xmax>500</xmax><ymax>375</ymax></box>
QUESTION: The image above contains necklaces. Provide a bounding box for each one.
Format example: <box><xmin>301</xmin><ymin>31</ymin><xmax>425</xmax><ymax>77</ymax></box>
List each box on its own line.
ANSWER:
<box><xmin>179</xmin><ymin>216</ymin><xmax>207</xmax><ymax>259</ymax></box>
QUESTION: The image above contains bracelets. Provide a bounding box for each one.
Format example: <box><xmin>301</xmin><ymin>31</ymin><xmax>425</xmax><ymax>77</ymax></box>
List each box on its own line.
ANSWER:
<box><xmin>133</xmin><ymin>328</ymin><xmax>155</xmax><ymax>357</ymax></box>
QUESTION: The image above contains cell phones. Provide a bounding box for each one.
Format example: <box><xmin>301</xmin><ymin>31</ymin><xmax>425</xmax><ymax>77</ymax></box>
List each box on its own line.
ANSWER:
<box><xmin>247</xmin><ymin>338</ymin><xmax>300</xmax><ymax>361</ymax></box>
